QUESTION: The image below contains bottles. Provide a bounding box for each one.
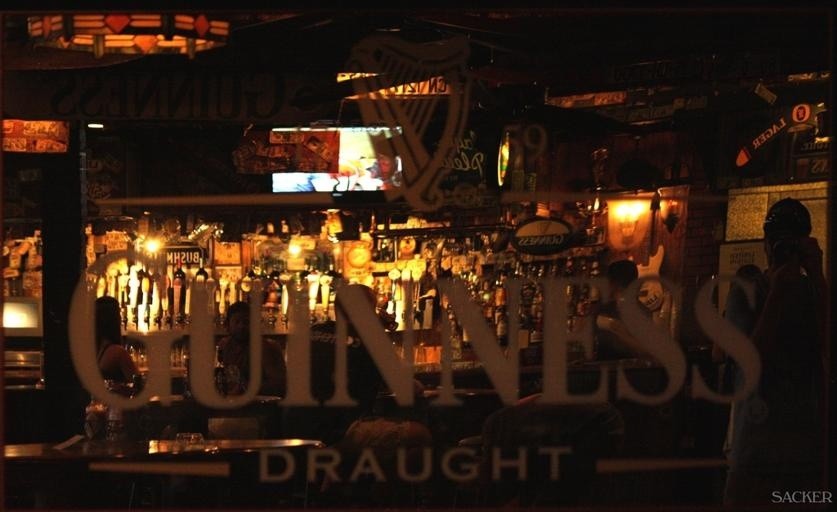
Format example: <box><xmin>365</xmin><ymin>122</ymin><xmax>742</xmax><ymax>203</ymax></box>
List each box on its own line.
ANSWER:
<box><xmin>169</xmin><ymin>257</ymin><xmax>209</xmax><ymax>321</ymax></box>
<box><xmin>83</xmin><ymin>393</ymin><xmax>126</xmax><ymax>463</ymax></box>
<box><xmin>368</xmin><ymin>203</ymin><xmax>606</xmax><ymax>350</ymax></box>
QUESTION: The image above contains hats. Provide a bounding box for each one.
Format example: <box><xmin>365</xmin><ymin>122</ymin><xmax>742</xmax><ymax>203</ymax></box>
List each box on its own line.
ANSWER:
<box><xmin>763</xmin><ymin>197</ymin><xmax>811</xmax><ymax>235</ymax></box>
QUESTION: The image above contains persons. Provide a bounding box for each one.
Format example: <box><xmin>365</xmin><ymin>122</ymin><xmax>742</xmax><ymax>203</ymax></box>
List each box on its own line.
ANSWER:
<box><xmin>81</xmin><ymin>196</ymin><xmax>834</xmax><ymax>510</ymax></box>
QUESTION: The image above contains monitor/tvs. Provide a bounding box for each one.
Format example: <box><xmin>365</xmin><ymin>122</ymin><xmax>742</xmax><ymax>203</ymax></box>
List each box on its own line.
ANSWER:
<box><xmin>269</xmin><ymin>122</ymin><xmax>405</xmax><ymax>194</ymax></box>
